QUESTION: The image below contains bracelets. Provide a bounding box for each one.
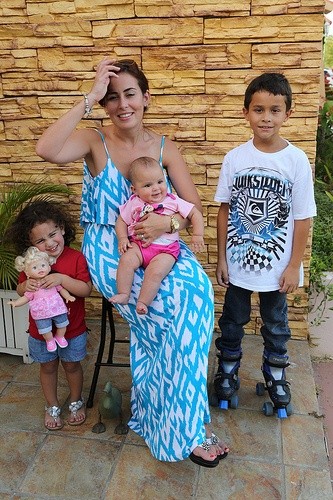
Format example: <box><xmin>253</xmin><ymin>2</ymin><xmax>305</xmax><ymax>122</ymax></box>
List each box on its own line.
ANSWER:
<box><xmin>83</xmin><ymin>94</ymin><xmax>93</xmax><ymax>118</ymax></box>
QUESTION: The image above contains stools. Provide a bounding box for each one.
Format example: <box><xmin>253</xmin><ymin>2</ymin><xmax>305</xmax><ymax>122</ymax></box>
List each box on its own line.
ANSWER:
<box><xmin>86</xmin><ymin>297</ymin><xmax>134</xmax><ymax>409</ymax></box>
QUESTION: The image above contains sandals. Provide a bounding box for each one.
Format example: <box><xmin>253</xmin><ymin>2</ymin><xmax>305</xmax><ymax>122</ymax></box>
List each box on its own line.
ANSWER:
<box><xmin>45</xmin><ymin>399</ymin><xmax>65</xmax><ymax>430</ymax></box>
<box><xmin>67</xmin><ymin>394</ymin><xmax>86</xmax><ymax>426</ymax></box>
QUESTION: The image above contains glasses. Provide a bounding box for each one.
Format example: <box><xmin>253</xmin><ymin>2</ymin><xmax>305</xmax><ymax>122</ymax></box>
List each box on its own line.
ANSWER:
<box><xmin>107</xmin><ymin>59</ymin><xmax>140</xmax><ymax>72</ymax></box>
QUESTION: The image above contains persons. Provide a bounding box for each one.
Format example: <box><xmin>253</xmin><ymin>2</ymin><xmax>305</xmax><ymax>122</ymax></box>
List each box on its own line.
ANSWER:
<box><xmin>108</xmin><ymin>157</ymin><xmax>204</xmax><ymax>314</ymax></box>
<box><xmin>210</xmin><ymin>73</ymin><xmax>318</xmax><ymax>418</ymax></box>
<box><xmin>34</xmin><ymin>59</ymin><xmax>230</xmax><ymax>467</ymax></box>
<box><xmin>6</xmin><ymin>246</ymin><xmax>76</xmax><ymax>352</ymax></box>
<box><xmin>10</xmin><ymin>202</ymin><xmax>94</xmax><ymax>431</ymax></box>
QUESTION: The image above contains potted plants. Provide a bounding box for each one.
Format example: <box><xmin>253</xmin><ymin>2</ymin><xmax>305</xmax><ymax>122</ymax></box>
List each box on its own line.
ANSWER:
<box><xmin>0</xmin><ymin>172</ymin><xmax>76</xmax><ymax>364</ymax></box>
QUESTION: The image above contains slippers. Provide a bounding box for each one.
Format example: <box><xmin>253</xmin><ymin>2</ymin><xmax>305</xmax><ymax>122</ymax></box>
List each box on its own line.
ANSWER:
<box><xmin>189</xmin><ymin>431</ymin><xmax>229</xmax><ymax>468</ymax></box>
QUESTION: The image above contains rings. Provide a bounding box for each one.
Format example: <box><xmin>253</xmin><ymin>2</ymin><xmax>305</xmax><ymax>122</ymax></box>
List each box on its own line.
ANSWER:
<box><xmin>141</xmin><ymin>235</ymin><xmax>143</xmax><ymax>239</ymax></box>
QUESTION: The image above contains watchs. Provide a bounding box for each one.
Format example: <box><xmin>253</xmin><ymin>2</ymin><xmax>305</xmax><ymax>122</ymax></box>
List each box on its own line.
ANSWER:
<box><xmin>166</xmin><ymin>215</ymin><xmax>180</xmax><ymax>234</ymax></box>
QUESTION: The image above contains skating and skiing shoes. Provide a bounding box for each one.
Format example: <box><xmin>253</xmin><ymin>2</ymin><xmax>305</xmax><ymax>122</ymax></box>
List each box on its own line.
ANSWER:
<box><xmin>256</xmin><ymin>345</ymin><xmax>293</xmax><ymax>418</ymax></box>
<box><xmin>211</xmin><ymin>351</ymin><xmax>243</xmax><ymax>410</ymax></box>
